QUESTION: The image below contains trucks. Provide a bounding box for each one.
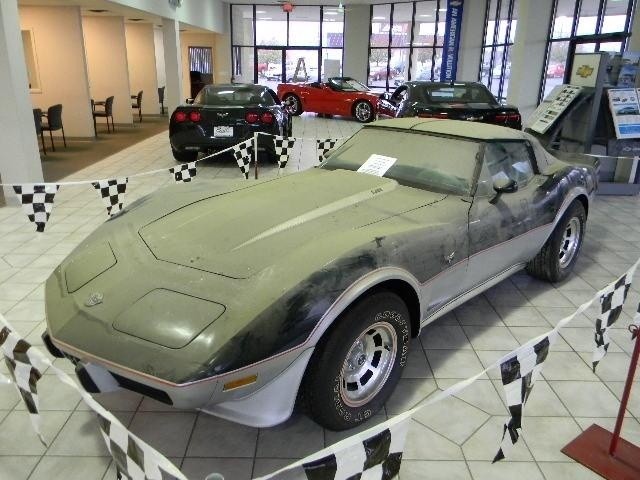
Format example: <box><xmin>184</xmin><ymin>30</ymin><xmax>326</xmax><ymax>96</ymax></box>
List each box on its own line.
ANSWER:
<box><xmin>265</xmin><ymin>63</ymin><xmax>281</xmax><ymax>81</ymax></box>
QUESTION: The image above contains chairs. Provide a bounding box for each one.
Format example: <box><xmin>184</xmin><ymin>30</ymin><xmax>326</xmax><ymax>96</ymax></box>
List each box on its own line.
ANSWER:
<box><xmin>191</xmin><ymin>71</ymin><xmax>201</xmax><ymax>86</ymax></box>
<box><xmin>131</xmin><ymin>90</ymin><xmax>143</xmax><ymax>122</ymax></box>
<box><xmin>193</xmin><ymin>73</ymin><xmax>213</xmax><ymax>99</ymax></box>
<box><xmin>42</xmin><ymin>104</ymin><xmax>67</xmax><ymax>151</ymax></box>
<box><xmin>92</xmin><ymin>96</ymin><xmax>116</xmax><ymax>132</ymax></box>
<box><xmin>33</xmin><ymin>109</ymin><xmax>47</xmax><ymax>155</ymax></box>
<box><xmin>158</xmin><ymin>85</ymin><xmax>165</xmax><ymax>114</ymax></box>
<box><xmin>250</xmin><ymin>95</ymin><xmax>265</xmax><ymax>104</ymax></box>
<box><xmin>91</xmin><ymin>99</ymin><xmax>94</xmax><ymax>113</ymax></box>
<box><xmin>206</xmin><ymin>95</ymin><xmax>220</xmax><ymax>105</ymax></box>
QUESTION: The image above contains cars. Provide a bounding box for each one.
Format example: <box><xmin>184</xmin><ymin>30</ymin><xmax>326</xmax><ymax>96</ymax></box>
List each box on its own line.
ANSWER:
<box><xmin>415</xmin><ymin>72</ymin><xmax>438</xmax><ymax>81</ymax></box>
<box><xmin>492</xmin><ymin>65</ymin><xmax>511</xmax><ymax>78</ymax></box>
<box><xmin>286</xmin><ymin>71</ymin><xmax>311</xmax><ymax>82</ymax></box>
<box><xmin>369</xmin><ymin>66</ymin><xmax>396</xmax><ymax>80</ymax></box>
<box><xmin>544</xmin><ymin>65</ymin><xmax>564</xmax><ymax>78</ymax></box>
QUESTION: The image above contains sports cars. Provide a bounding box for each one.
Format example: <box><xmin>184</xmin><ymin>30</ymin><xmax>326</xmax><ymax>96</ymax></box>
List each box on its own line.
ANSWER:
<box><xmin>42</xmin><ymin>117</ymin><xmax>602</xmax><ymax>432</ymax></box>
<box><xmin>169</xmin><ymin>82</ymin><xmax>293</xmax><ymax>164</ymax></box>
<box><xmin>377</xmin><ymin>80</ymin><xmax>523</xmax><ymax>132</ymax></box>
<box><xmin>276</xmin><ymin>76</ymin><xmax>380</xmax><ymax>123</ymax></box>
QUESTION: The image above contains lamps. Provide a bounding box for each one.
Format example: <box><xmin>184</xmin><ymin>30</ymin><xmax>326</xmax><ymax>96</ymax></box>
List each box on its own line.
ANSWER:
<box><xmin>281</xmin><ymin>1</ymin><xmax>293</xmax><ymax>13</ymax></box>
<box><xmin>338</xmin><ymin>0</ymin><xmax>342</xmax><ymax>9</ymax></box>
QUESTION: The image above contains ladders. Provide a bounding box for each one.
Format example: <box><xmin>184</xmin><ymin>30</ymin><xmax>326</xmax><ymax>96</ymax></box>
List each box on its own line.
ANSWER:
<box><xmin>293</xmin><ymin>58</ymin><xmax>308</xmax><ymax>82</ymax></box>
